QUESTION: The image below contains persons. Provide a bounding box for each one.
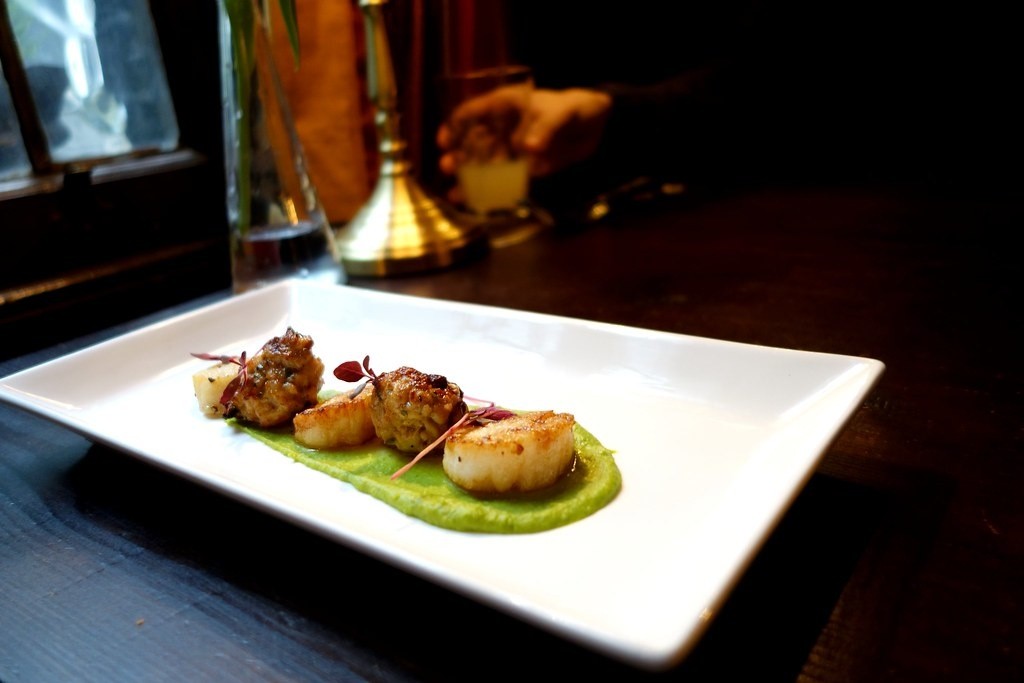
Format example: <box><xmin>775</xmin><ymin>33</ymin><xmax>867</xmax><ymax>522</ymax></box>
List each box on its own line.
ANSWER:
<box><xmin>418</xmin><ymin>1</ymin><xmax>1024</xmax><ymax>212</ymax></box>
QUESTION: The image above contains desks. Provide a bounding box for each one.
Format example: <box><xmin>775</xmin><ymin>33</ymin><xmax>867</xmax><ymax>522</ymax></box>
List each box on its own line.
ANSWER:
<box><xmin>0</xmin><ymin>224</ymin><xmax>1024</xmax><ymax>683</ymax></box>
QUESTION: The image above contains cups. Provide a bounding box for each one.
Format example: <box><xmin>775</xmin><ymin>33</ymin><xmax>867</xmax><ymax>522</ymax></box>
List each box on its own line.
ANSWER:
<box><xmin>444</xmin><ymin>64</ymin><xmax>544</xmax><ymax>248</ymax></box>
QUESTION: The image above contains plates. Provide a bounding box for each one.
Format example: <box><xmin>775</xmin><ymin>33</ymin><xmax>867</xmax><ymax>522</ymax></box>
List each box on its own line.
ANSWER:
<box><xmin>0</xmin><ymin>274</ymin><xmax>888</xmax><ymax>671</ymax></box>
